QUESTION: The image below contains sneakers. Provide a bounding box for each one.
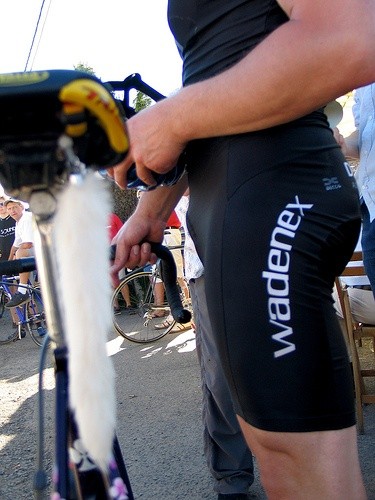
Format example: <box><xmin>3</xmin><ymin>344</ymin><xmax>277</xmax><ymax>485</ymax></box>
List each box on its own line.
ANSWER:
<box><xmin>8</xmin><ymin>329</ymin><xmax>26</xmax><ymax>339</ymax></box>
<box><xmin>5</xmin><ymin>289</ymin><xmax>31</xmax><ymax>309</ymax></box>
<box><xmin>36</xmin><ymin>325</ymin><xmax>45</xmax><ymax>336</ymax></box>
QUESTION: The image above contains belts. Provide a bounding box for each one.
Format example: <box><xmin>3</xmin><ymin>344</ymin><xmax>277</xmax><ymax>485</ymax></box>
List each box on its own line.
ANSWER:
<box><xmin>346</xmin><ymin>285</ymin><xmax>372</xmax><ymax>291</ymax></box>
<box><xmin>165</xmin><ymin>226</ymin><xmax>178</xmax><ymax>229</ymax></box>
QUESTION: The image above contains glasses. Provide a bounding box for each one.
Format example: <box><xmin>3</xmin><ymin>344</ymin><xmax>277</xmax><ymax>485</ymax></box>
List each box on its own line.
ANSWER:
<box><xmin>0</xmin><ymin>203</ymin><xmax>3</xmax><ymax>207</ymax></box>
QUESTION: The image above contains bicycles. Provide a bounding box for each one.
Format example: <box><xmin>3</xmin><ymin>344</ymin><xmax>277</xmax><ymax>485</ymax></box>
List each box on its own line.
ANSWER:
<box><xmin>0</xmin><ymin>274</ymin><xmax>50</xmax><ymax>349</ymax></box>
<box><xmin>111</xmin><ymin>230</ymin><xmax>192</xmax><ymax>344</ymax></box>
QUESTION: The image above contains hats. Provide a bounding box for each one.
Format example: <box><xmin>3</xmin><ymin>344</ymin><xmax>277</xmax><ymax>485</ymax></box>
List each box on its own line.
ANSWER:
<box><xmin>3</xmin><ymin>197</ymin><xmax>21</xmax><ymax>206</ymax></box>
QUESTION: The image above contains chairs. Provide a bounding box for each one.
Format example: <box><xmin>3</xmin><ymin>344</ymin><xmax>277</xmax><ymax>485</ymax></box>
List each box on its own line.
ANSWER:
<box><xmin>334</xmin><ymin>251</ymin><xmax>375</xmax><ymax>434</ymax></box>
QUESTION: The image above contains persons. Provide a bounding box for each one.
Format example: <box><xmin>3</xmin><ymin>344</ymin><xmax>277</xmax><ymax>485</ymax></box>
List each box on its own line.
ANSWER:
<box><xmin>105</xmin><ymin>0</ymin><xmax>375</xmax><ymax>500</ymax></box>
<box><xmin>0</xmin><ymin>82</ymin><xmax>375</xmax><ymax>500</ymax></box>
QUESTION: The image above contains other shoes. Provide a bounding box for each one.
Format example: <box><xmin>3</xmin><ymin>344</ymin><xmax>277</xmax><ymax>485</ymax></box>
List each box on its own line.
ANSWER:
<box><xmin>127</xmin><ymin>306</ymin><xmax>136</xmax><ymax>315</ymax></box>
<box><xmin>154</xmin><ymin>320</ymin><xmax>174</xmax><ymax>328</ymax></box>
<box><xmin>169</xmin><ymin>321</ymin><xmax>191</xmax><ymax>332</ymax></box>
<box><xmin>114</xmin><ymin>308</ymin><xmax>121</xmax><ymax>315</ymax></box>
